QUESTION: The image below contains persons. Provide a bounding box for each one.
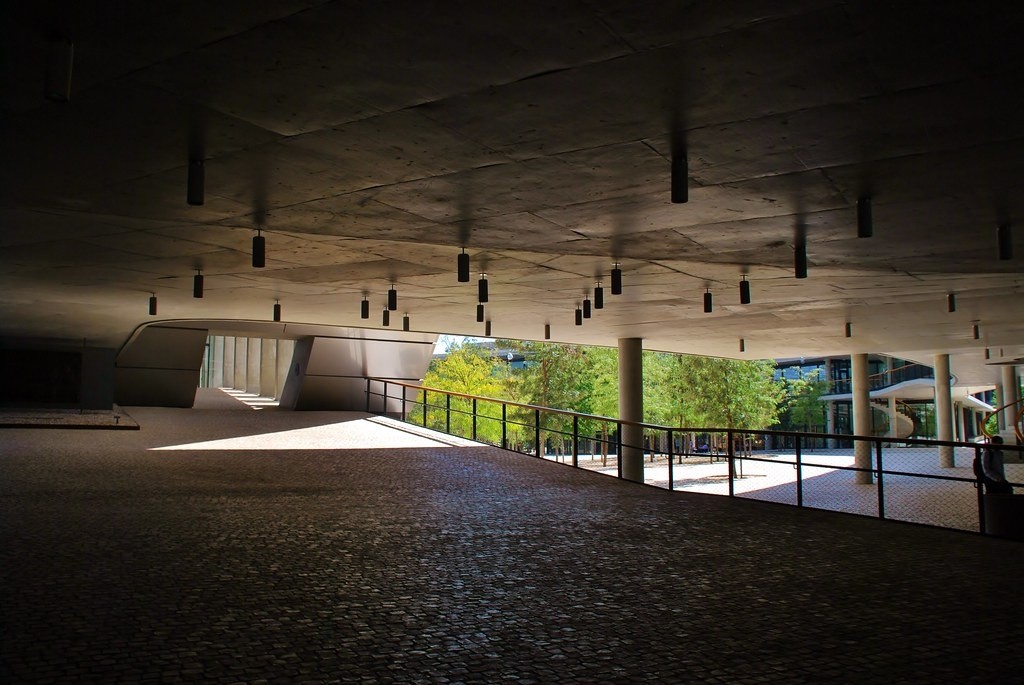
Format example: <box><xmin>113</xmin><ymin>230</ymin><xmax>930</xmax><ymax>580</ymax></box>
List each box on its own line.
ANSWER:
<box><xmin>980</xmin><ymin>436</ymin><xmax>1006</xmax><ymax>496</ymax></box>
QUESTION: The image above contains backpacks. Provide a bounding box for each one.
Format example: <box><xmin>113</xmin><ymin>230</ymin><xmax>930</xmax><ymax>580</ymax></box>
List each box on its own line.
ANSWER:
<box><xmin>973</xmin><ymin>450</ymin><xmax>1003</xmax><ymax>479</ymax></box>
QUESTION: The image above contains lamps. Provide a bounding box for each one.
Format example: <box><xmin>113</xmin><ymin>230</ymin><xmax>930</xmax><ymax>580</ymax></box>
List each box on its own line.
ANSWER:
<box><xmin>670</xmin><ymin>143</ymin><xmax>1015</xmax><ymax>359</ymax></box>
<box><xmin>383</xmin><ymin>306</ymin><xmax>389</xmax><ymax>326</ymax></box>
<box><xmin>388</xmin><ymin>284</ymin><xmax>397</xmax><ymax>310</ymax></box>
<box><xmin>594</xmin><ymin>282</ymin><xmax>603</xmax><ymax>309</ymax></box>
<box><xmin>361</xmin><ymin>296</ymin><xmax>369</xmax><ymax>318</ymax></box>
<box><xmin>457</xmin><ymin>247</ymin><xmax>469</xmax><ymax>282</ymax></box>
<box><xmin>575</xmin><ymin>302</ymin><xmax>582</xmax><ymax>325</ymax></box>
<box><xmin>610</xmin><ymin>262</ymin><xmax>622</xmax><ymax>295</ymax></box>
<box><xmin>149</xmin><ymin>292</ymin><xmax>157</xmax><ymax>316</ymax></box>
<box><xmin>478</xmin><ymin>273</ymin><xmax>488</xmax><ymax>303</ymax></box>
<box><xmin>186</xmin><ymin>157</ymin><xmax>205</xmax><ymax>206</ymax></box>
<box><xmin>583</xmin><ymin>293</ymin><xmax>591</xmax><ymax>318</ymax></box>
<box><xmin>194</xmin><ymin>269</ymin><xmax>204</xmax><ymax>298</ymax></box>
<box><xmin>477</xmin><ymin>302</ymin><xmax>550</xmax><ymax>340</ymax></box>
<box><xmin>252</xmin><ymin>229</ymin><xmax>266</xmax><ymax>268</ymax></box>
<box><xmin>274</xmin><ymin>299</ymin><xmax>280</xmax><ymax>321</ymax></box>
<box><xmin>403</xmin><ymin>312</ymin><xmax>409</xmax><ymax>331</ymax></box>
